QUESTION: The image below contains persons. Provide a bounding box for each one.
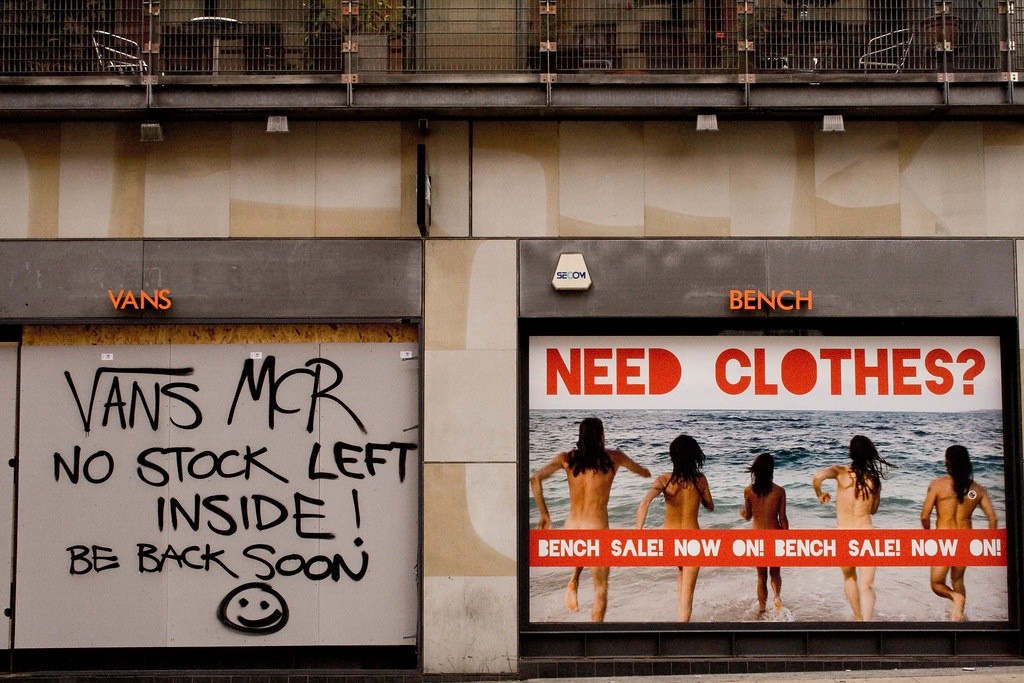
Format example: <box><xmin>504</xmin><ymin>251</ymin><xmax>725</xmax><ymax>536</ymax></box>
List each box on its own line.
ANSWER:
<box><xmin>919</xmin><ymin>445</ymin><xmax>999</xmax><ymax>620</ymax></box>
<box><xmin>812</xmin><ymin>435</ymin><xmax>899</xmax><ymax>621</ymax></box>
<box><xmin>636</xmin><ymin>434</ymin><xmax>716</xmax><ymax>622</ymax></box>
<box><xmin>530</xmin><ymin>416</ymin><xmax>651</xmax><ymax>623</ymax></box>
<box><xmin>737</xmin><ymin>452</ymin><xmax>791</xmax><ymax>614</ymax></box>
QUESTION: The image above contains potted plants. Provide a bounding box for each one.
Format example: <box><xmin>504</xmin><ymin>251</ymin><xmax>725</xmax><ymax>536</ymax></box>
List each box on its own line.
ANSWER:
<box><xmin>304</xmin><ymin>1</ymin><xmax>419</xmax><ymax>75</ymax></box>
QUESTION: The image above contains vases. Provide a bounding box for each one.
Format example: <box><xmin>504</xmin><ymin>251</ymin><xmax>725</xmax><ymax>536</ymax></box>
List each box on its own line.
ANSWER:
<box><xmin>920</xmin><ymin>14</ymin><xmax>963</xmax><ymax>47</ymax></box>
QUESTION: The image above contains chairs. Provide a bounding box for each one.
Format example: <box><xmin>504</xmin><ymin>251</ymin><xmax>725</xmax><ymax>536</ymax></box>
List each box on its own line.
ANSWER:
<box><xmin>91</xmin><ymin>29</ymin><xmax>148</xmax><ymax>76</ymax></box>
<box><xmin>859</xmin><ymin>26</ymin><xmax>916</xmax><ymax>75</ymax></box>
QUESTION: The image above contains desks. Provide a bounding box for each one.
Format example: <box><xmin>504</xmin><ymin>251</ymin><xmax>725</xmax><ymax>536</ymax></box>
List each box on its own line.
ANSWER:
<box><xmin>781</xmin><ymin>20</ymin><xmax>844</xmax><ymax>71</ymax></box>
<box><xmin>181</xmin><ymin>16</ymin><xmax>242</xmax><ymax>75</ymax></box>
